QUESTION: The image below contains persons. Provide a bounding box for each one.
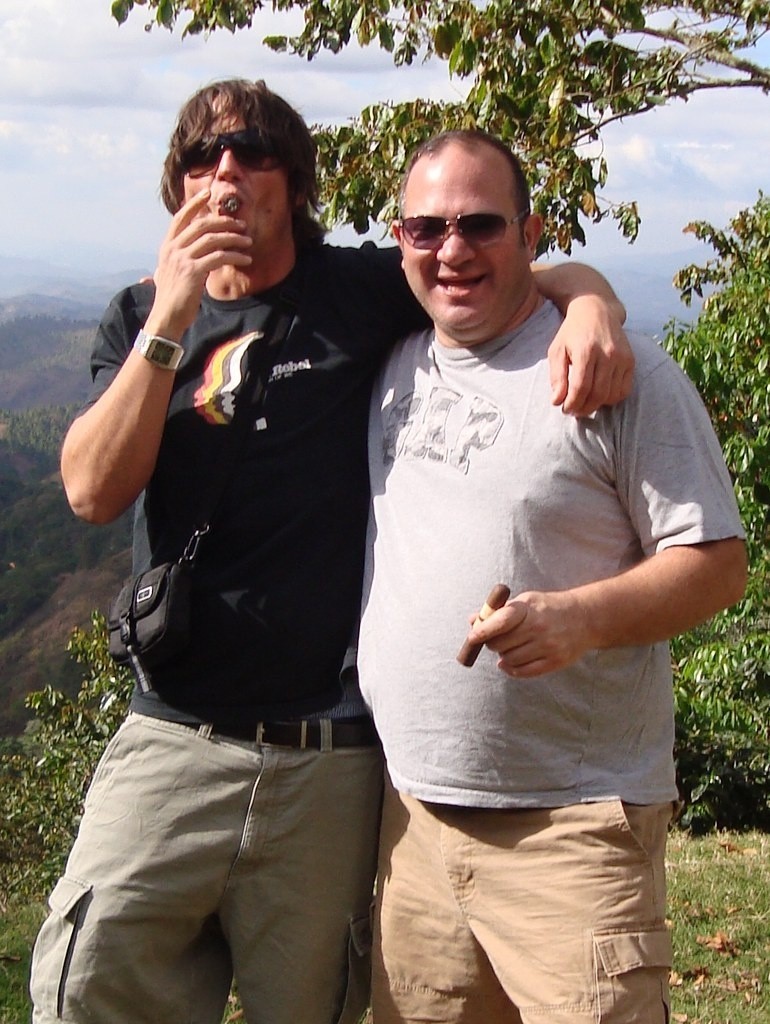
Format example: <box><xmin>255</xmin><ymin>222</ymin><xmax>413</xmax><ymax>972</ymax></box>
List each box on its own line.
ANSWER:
<box><xmin>27</xmin><ymin>74</ymin><xmax>641</xmax><ymax>1024</ymax></box>
<box><xmin>356</xmin><ymin>133</ymin><xmax>753</xmax><ymax>1024</ymax></box>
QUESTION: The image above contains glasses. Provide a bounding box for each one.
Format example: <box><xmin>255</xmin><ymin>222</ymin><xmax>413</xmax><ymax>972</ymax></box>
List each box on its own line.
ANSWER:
<box><xmin>398</xmin><ymin>208</ymin><xmax>531</xmax><ymax>250</ymax></box>
<box><xmin>180</xmin><ymin>125</ymin><xmax>285</xmax><ymax>178</ymax></box>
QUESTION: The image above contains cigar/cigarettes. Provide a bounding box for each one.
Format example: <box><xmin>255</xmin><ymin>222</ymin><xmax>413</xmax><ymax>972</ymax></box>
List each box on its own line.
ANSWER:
<box><xmin>456</xmin><ymin>583</ymin><xmax>510</xmax><ymax>668</ymax></box>
<box><xmin>225</xmin><ymin>200</ymin><xmax>239</xmax><ymax>211</ymax></box>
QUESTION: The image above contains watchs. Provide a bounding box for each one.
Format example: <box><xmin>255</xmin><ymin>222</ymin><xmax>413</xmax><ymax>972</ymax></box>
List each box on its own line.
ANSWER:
<box><xmin>135</xmin><ymin>331</ymin><xmax>184</xmax><ymax>371</ymax></box>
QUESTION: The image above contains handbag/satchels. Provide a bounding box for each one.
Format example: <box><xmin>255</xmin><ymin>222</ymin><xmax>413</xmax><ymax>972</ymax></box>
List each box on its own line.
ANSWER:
<box><xmin>107</xmin><ymin>561</ymin><xmax>193</xmax><ymax>695</ymax></box>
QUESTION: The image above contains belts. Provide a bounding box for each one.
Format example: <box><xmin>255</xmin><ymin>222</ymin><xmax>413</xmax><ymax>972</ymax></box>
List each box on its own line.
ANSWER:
<box><xmin>129</xmin><ymin>684</ymin><xmax>382</xmax><ymax>750</ymax></box>
